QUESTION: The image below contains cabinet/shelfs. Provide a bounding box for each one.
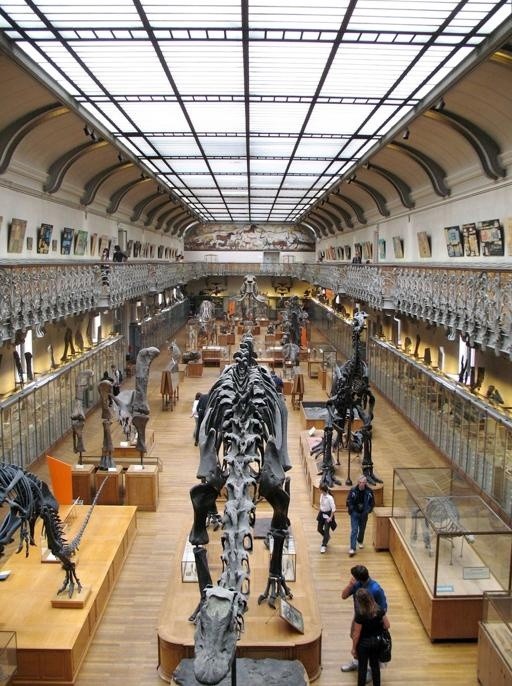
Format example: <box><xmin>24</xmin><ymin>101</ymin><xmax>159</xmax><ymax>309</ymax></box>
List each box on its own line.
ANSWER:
<box><xmin>180</xmin><ymin>536</ymin><xmax>203</xmax><ymax>582</ymax></box>
<box><xmin>308</xmin><ymin>346</ymin><xmax>323</xmax><ymax>378</ymax></box>
<box><xmin>318</xmin><ymin>345</ymin><xmax>337</xmax><ymax>391</ymax></box>
<box><xmin>474</xmin><ymin>590</ymin><xmax>512</xmax><ymax>686</ymax></box>
<box><xmin>220</xmin><ymin>346</ymin><xmax>231</xmax><ymax>375</ymax></box>
<box><xmin>0</xmin><ymin>629</ymin><xmax>19</xmax><ymax>686</ymax></box>
<box><xmin>326</xmin><ymin>361</ymin><xmax>340</xmax><ymax>398</ymax></box>
<box><xmin>269</xmin><ymin>533</ymin><xmax>297</xmax><ymax>583</ymax></box>
<box><xmin>388</xmin><ymin>465</ymin><xmax>512</xmax><ymax>644</ymax></box>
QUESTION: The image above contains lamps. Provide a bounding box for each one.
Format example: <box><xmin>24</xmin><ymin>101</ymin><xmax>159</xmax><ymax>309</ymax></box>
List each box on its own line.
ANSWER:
<box><xmin>84</xmin><ymin>123</ymin><xmax>91</xmax><ymax>136</ymax></box>
<box><xmin>141</xmin><ymin>173</ymin><xmax>146</xmax><ymax>180</ymax></box>
<box><xmin>118</xmin><ymin>151</ymin><xmax>123</xmax><ymax>161</ymax></box>
<box><xmin>91</xmin><ymin>128</ymin><xmax>97</xmax><ymax>141</ymax></box>
<box><xmin>403</xmin><ymin>127</ymin><xmax>409</xmax><ymax>139</ymax></box>
<box><xmin>436</xmin><ymin>101</ymin><xmax>445</xmax><ymax>111</ymax></box>
<box><xmin>351</xmin><ymin>173</ymin><xmax>357</xmax><ymax>181</ymax></box>
<box><xmin>365</xmin><ymin>161</ymin><xmax>369</xmax><ymax>170</ymax></box>
<box><xmin>346</xmin><ymin>176</ymin><xmax>352</xmax><ymax>184</ymax></box>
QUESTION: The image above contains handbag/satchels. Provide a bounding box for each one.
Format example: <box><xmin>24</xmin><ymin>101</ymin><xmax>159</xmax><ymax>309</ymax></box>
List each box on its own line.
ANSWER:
<box><xmin>377</xmin><ymin>629</ymin><xmax>391</xmax><ymax>663</ymax></box>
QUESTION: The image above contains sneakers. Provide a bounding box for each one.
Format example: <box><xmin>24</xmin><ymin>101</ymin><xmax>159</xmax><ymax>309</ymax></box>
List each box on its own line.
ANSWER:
<box><xmin>348</xmin><ymin>550</ymin><xmax>354</xmax><ymax>555</ymax></box>
<box><xmin>342</xmin><ymin>663</ymin><xmax>358</xmax><ymax>672</ymax></box>
<box><xmin>357</xmin><ymin>542</ymin><xmax>364</xmax><ymax>548</ymax></box>
<box><xmin>320</xmin><ymin>547</ymin><xmax>325</xmax><ymax>553</ymax></box>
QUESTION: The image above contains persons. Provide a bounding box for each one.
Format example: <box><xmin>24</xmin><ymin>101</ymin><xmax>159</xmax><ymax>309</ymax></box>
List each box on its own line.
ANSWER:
<box><xmin>190</xmin><ymin>391</ymin><xmax>202</xmax><ymax>437</ymax></box>
<box><xmin>268</xmin><ymin>371</ymin><xmax>283</xmax><ymax>398</ymax></box>
<box><xmin>114</xmin><ymin>245</ymin><xmax>128</xmax><ymax>263</ymax></box>
<box><xmin>318</xmin><ymin>256</ymin><xmax>325</xmax><ymax>261</ymax></box>
<box><xmin>100</xmin><ymin>373</ymin><xmax>116</xmax><ymax>407</ymax></box>
<box><xmin>101</xmin><ymin>248</ymin><xmax>112</xmax><ymax>287</ymax></box>
<box><xmin>346</xmin><ymin>477</ymin><xmax>375</xmax><ymax>556</ymax></box>
<box><xmin>187</xmin><ymin>311</ymin><xmax>195</xmax><ymax>319</ymax></box>
<box><xmin>174</xmin><ymin>254</ymin><xmax>181</xmax><ymax>262</ymax></box>
<box><xmin>352</xmin><ymin>251</ymin><xmax>361</xmax><ymax>264</ymax></box>
<box><xmin>195</xmin><ymin>393</ymin><xmax>209</xmax><ymax>446</ymax></box>
<box><xmin>316</xmin><ymin>485</ymin><xmax>337</xmax><ymax>554</ymax></box>
<box><xmin>109</xmin><ymin>365</ymin><xmax>121</xmax><ymax>397</ymax></box>
<box><xmin>340</xmin><ymin>566</ymin><xmax>388</xmax><ymax>682</ymax></box>
<box><xmin>226</xmin><ymin>311</ymin><xmax>230</xmax><ymax>323</ymax></box>
<box><xmin>179</xmin><ymin>256</ymin><xmax>184</xmax><ymax>262</ymax></box>
<box><xmin>351</xmin><ymin>589</ymin><xmax>391</xmax><ymax>686</ymax></box>
